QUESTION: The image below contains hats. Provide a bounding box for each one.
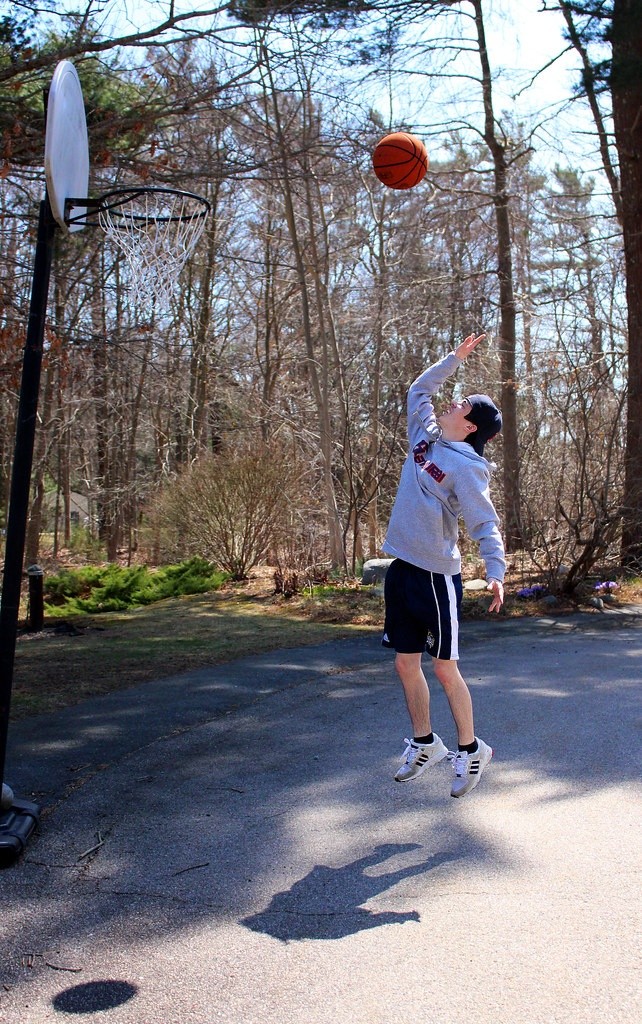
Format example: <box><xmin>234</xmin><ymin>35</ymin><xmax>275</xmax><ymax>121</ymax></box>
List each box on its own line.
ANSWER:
<box><xmin>463</xmin><ymin>394</ymin><xmax>503</xmax><ymax>457</ymax></box>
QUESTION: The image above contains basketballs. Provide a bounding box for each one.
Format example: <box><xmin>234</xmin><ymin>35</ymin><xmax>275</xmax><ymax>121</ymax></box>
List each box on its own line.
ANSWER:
<box><xmin>372</xmin><ymin>132</ymin><xmax>429</xmax><ymax>190</ymax></box>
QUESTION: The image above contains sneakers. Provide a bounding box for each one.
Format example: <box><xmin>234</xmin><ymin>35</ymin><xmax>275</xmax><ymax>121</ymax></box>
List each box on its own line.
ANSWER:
<box><xmin>445</xmin><ymin>736</ymin><xmax>494</xmax><ymax>798</ymax></box>
<box><xmin>395</xmin><ymin>733</ymin><xmax>448</xmax><ymax>782</ymax></box>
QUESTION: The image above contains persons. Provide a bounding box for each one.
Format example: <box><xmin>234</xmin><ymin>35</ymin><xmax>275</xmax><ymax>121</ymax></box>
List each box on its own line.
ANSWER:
<box><xmin>382</xmin><ymin>333</ymin><xmax>506</xmax><ymax>797</ymax></box>
